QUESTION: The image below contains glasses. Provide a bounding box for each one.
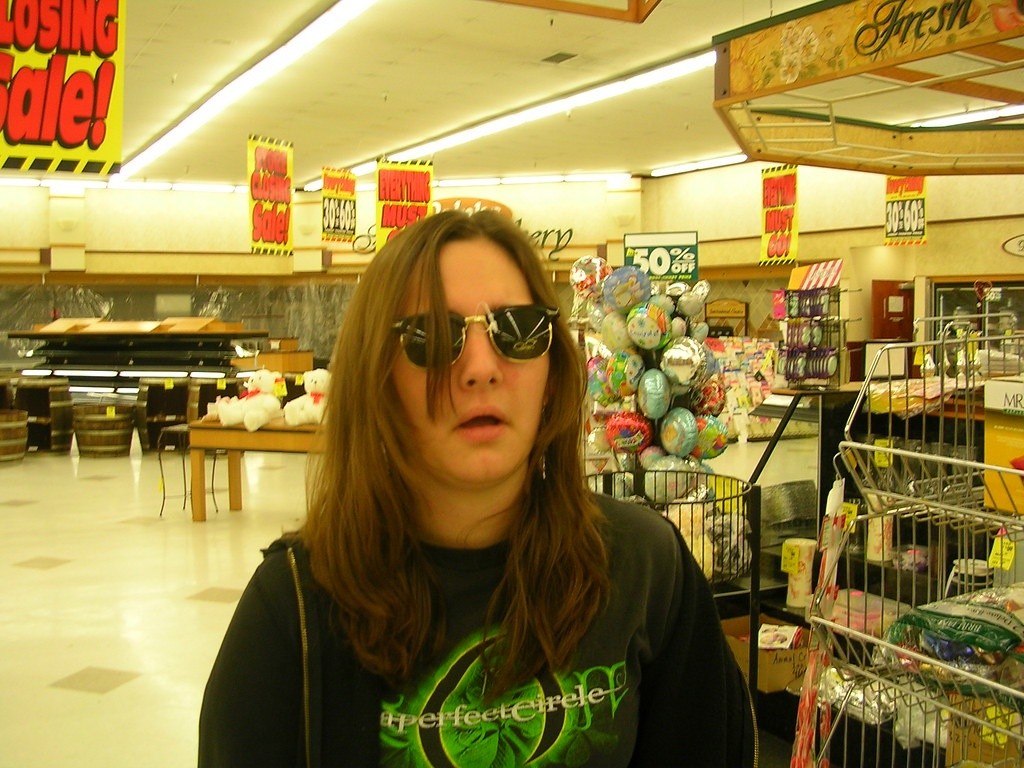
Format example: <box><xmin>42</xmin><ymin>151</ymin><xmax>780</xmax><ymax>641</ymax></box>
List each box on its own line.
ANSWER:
<box><xmin>391</xmin><ymin>306</ymin><xmax>560</xmax><ymax>369</ymax></box>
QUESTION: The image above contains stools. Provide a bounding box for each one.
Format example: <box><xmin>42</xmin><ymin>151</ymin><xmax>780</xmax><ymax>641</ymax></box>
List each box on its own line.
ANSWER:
<box><xmin>158</xmin><ymin>424</ymin><xmax>218</xmax><ymax>516</ymax></box>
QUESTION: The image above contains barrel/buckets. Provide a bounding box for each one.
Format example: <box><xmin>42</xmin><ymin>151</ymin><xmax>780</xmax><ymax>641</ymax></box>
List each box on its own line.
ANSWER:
<box><xmin>1</xmin><ymin>377</ymin><xmax>73</xmax><ymax>454</ymax></box>
<box><xmin>0</xmin><ymin>408</ymin><xmax>28</xmax><ymax>461</ymax></box>
<box><xmin>136</xmin><ymin>375</ymin><xmax>238</xmax><ymax>454</ymax></box>
<box><xmin>72</xmin><ymin>402</ymin><xmax>136</xmax><ymax>458</ymax></box>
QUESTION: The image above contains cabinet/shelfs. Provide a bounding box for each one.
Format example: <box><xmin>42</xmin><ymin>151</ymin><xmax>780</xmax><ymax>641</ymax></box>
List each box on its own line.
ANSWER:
<box><xmin>725</xmin><ymin>389</ymin><xmax>988</xmax><ymax>639</ymax></box>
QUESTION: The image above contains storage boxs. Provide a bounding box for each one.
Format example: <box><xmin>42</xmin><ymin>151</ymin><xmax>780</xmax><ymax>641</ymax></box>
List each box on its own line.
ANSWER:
<box><xmin>721</xmin><ymin>614</ymin><xmax>834</xmax><ymax>693</ymax></box>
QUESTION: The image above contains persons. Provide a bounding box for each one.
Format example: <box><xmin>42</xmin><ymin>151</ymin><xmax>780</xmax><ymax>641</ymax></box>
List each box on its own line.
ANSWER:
<box><xmin>198</xmin><ymin>208</ymin><xmax>759</xmax><ymax>768</ymax></box>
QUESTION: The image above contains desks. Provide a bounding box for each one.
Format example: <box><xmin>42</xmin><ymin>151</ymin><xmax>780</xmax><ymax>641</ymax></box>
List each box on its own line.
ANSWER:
<box><xmin>189</xmin><ymin>420</ymin><xmax>326</xmax><ymax>523</ymax></box>
